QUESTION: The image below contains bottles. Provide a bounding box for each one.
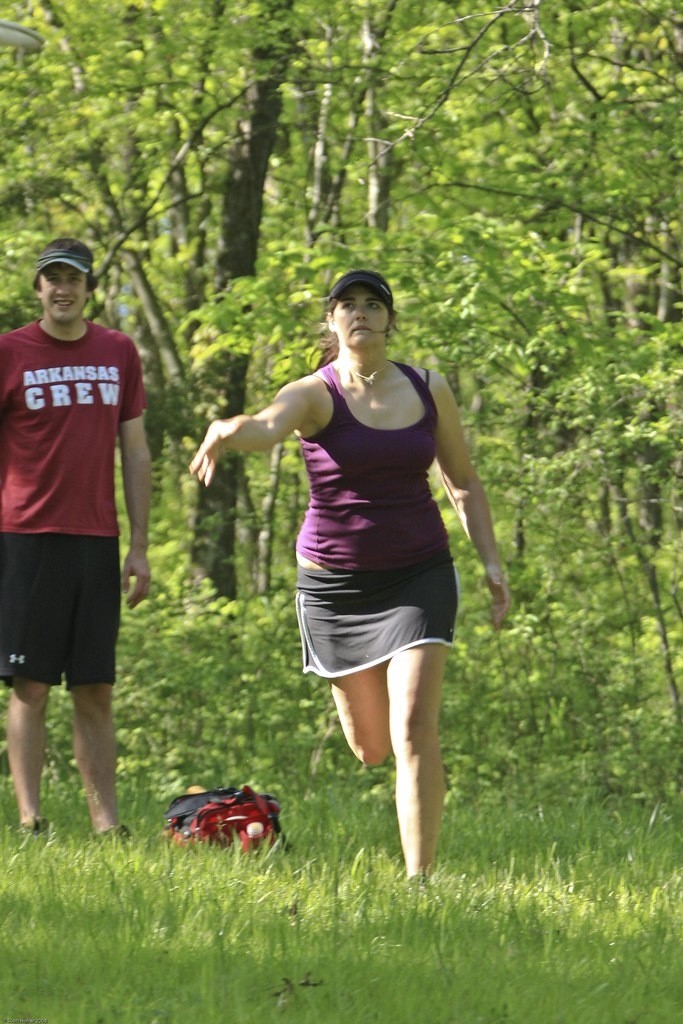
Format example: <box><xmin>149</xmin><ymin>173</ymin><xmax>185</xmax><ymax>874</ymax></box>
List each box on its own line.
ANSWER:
<box><xmin>244</xmin><ymin>821</ymin><xmax>265</xmax><ymax>858</ymax></box>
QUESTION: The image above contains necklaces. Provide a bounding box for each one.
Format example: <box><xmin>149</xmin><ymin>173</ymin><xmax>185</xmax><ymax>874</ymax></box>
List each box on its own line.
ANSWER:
<box><xmin>350</xmin><ymin>364</ymin><xmax>385</xmax><ymax>386</ymax></box>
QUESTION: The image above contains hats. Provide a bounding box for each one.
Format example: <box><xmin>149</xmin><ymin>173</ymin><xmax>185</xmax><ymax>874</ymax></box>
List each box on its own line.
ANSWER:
<box><xmin>329</xmin><ymin>272</ymin><xmax>392</xmax><ymax>305</ymax></box>
<box><xmin>35</xmin><ymin>248</ymin><xmax>93</xmax><ymax>273</ymax></box>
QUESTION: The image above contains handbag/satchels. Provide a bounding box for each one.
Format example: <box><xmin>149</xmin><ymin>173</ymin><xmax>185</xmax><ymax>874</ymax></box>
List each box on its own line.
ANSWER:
<box><xmin>165</xmin><ymin>786</ymin><xmax>293</xmax><ymax>854</ymax></box>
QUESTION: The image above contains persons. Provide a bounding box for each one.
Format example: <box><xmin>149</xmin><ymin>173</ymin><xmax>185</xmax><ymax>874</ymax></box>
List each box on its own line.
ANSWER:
<box><xmin>0</xmin><ymin>236</ymin><xmax>154</xmax><ymax>847</ymax></box>
<box><xmin>189</xmin><ymin>264</ymin><xmax>512</xmax><ymax>889</ymax></box>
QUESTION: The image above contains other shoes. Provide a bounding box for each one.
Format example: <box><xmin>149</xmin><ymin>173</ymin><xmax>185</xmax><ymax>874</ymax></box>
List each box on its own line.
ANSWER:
<box><xmin>21</xmin><ymin>816</ymin><xmax>49</xmax><ymax>837</ymax></box>
<box><xmin>103</xmin><ymin>824</ymin><xmax>131</xmax><ymax>846</ymax></box>
